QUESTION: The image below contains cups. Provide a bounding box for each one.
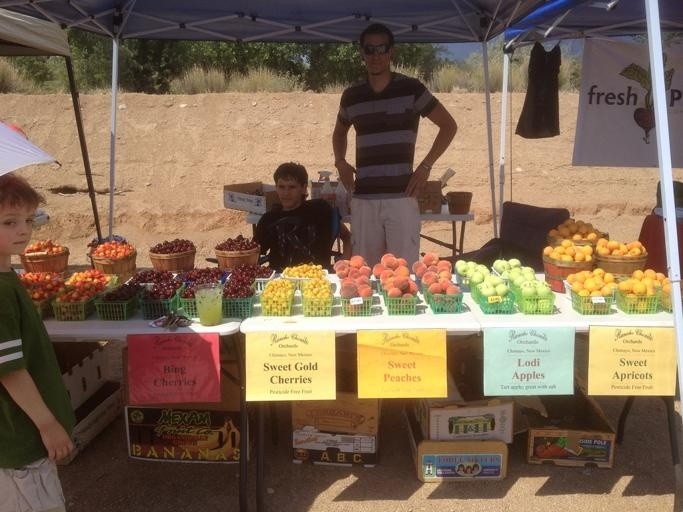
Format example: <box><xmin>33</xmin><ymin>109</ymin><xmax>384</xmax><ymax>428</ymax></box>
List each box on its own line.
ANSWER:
<box><xmin>192</xmin><ymin>283</ymin><xmax>224</xmax><ymax>325</ymax></box>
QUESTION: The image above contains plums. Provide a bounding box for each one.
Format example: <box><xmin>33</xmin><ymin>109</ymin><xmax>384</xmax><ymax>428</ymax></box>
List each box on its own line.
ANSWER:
<box><xmin>103</xmin><ymin>235</ymin><xmax>273</xmax><ymax>320</ymax></box>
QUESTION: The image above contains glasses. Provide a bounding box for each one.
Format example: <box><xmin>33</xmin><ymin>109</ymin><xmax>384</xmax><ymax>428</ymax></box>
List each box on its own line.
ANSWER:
<box><xmin>364</xmin><ymin>44</ymin><xmax>389</xmax><ymax>54</ymax></box>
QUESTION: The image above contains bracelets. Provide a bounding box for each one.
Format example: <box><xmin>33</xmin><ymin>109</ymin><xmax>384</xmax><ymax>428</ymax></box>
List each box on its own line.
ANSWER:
<box><xmin>420</xmin><ymin>162</ymin><xmax>431</xmax><ymax>170</ymax></box>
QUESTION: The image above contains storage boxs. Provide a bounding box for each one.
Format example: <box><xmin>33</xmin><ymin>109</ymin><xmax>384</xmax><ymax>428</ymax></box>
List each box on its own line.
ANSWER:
<box><xmin>53</xmin><ymin>376</ymin><xmax>124</xmax><ymax>469</ymax></box>
<box><xmin>223</xmin><ymin>178</ymin><xmax>289</xmax><ymax>214</ymax></box>
<box><xmin>406</xmin><ymin>352</ymin><xmax>514</xmax><ymax>442</ymax></box>
<box><xmin>289</xmin><ymin>386</ymin><xmax>385</xmax><ymax>435</ymax></box>
<box><xmin>410</xmin><ymin>170</ymin><xmax>454</xmax><ymax>214</ymax></box>
<box><xmin>291</xmin><ymin>436</ymin><xmax>384</xmax><ymax>467</ymax></box>
<box><xmin>128</xmin><ymin>406</ymin><xmax>247</xmax><ymax>464</ymax></box>
<box><xmin>400</xmin><ymin>409</ymin><xmax>510</xmax><ymax>480</ymax></box>
<box><xmin>122</xmin><ymin>360</ymin><xmax>244</xmax><ymax>412</ymax></box>
<box><xmin>312</xmin><ymin>179</ymin><xmax>352</xmax><ymax>211</ymax></box>
<box><xmin>525</xmin><ymin>369</ymin><xmax>615</xmax><ymax>468</ymax></box>
<box><xmin>57</xmin><ymin>347</ymin><xmax>108</xmax><ymax>411</ymax></box>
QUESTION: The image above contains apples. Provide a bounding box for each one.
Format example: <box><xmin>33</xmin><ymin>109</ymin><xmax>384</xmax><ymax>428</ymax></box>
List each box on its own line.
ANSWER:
<box><xmin>454</xmin><ymin>258</ymin><xmax>551</xmax><ymax>315</ymax></box>
<box><xmin>537</xmin><ymin>444</ymin><xmax>568</xmax><ymax>460</ymax></box>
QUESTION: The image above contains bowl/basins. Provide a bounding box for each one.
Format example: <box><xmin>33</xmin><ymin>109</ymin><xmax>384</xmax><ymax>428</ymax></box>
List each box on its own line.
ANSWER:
<box><xmin>19</xmin><ymin>248</ymin><xmax>70</xmax><ymax>274</ymax></box>
<box><xmin>149</xmin><ymin>248</ymin><xmax>196</xmax><ymax>272</ymax></box>
<box><xmin>88</xmin><ymin>250</ymin><xmax>138</xmax><ymax>275</ymax></box>
<box><xmin>213</xmin><ymin>244</ymin><xmax>262</xmax><ymax>270</ymax></box>
<box><xmin>546</xmin><ymin>234</ymin><xmax>610</xmax><ymax>252</ymax></box>
<box><xmin>593</xmin><ymin>250</ymin><xmax>649</xmax><ymax>275</ymax></box>
<box><xmin>540</xmin><ymin>251</ymin><xmax>596</xmax><ymax>293</ymax></box>
<box><xmin>447</xmin><ymin>192</ymin><xmax>473</xmax><ymax>214</ymax></box>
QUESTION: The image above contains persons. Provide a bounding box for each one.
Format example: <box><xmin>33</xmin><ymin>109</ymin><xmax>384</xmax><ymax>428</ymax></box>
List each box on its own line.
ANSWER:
<box><xmin>251</xmin><ymin>162</ymin><xmax>337</xmax><ymax>274</ymax></box>
<box><xmin>332</xmin><ymin>22</ymin><xmax>458</xmax><ymax>278</ymax></box>
<box><xmin>0</xmin><ymin>172</ymin><xmax>77</xmax><ymax>511</ymax></box>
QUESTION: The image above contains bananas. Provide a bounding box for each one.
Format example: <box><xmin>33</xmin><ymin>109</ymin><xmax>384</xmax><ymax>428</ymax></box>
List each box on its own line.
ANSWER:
<box><xmin>197</xmin><ymin>420</ymin><xmax>240</xmax><ymax>462</ymax></box>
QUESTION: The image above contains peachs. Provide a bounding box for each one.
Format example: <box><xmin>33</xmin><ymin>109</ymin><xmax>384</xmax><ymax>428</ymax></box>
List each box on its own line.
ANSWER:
<box><xmin>18</xmin><ymin>237</ymin><xmax>135</xmax><ymax>321</ymax></box>
<box><xmin>333</xmin><ymin>252</ymin><xmax>460</xmax><ymax>314</ymax></box>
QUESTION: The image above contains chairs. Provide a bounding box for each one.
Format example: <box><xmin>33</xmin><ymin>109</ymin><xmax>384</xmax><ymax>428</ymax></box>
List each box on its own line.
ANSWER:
<box><xmin>255</xmin><ymin>198</ymin><xmax>340</xmax><ymax>269</ymax></box>
<box><xmin>637</xmin><ymin>214</ymin><xmax>683</xmax><ymax>277</ymax></box>
<box><xmin>477</xmin><ymin>199</ymin><xmax>572</xmax><ymax>277</ymax></box>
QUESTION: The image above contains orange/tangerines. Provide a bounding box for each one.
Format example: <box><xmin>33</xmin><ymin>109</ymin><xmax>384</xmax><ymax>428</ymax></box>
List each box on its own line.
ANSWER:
<box><xmin>544</xmin><ymin>219</ymin><xmax>671</xmax><ymax>313</ymax></box>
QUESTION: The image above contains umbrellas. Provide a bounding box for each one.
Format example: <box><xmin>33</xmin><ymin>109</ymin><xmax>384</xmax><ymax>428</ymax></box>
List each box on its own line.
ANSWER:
<box><xmin>1</xmin><ymin>121</ymin><xmax>62</xmax><ymax>174</ymax></box>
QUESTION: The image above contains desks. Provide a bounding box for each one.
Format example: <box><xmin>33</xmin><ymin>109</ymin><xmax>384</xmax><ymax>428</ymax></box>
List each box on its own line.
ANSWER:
<box><xmin>247</xmin><ymin>206</ymin><xmax>473</xmax><ymax>258</ymax></box>
<box><xmin>25</xmin><ymin>291</ymin><xmax>683</xmax><ymax>467</ymax></box>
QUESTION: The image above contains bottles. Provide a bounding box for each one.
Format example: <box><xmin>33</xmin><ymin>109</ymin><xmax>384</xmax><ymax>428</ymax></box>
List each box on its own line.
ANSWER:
<box><xmin>335</xmin><ymin>177</ymin><xmax>348</xmax><ymax>216</ymax></box>
